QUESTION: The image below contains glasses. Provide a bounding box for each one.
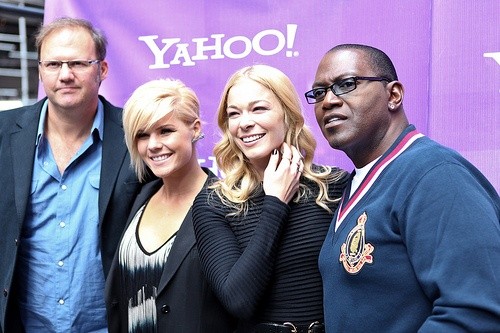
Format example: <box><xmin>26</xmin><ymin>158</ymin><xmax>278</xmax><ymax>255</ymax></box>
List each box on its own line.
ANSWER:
<box><xmin>305</xmin><ymin>75</ymin><xmax>391</xmax><ymax>104</ymax></box>
<box><xmin>37</xmin><ymin>59</ymin><xmax>100</xmax><ymax>72</ymax></box>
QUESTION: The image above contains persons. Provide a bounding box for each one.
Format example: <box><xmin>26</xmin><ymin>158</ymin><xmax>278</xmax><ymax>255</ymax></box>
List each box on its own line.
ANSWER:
<box><xmin>105</xmin><ymin>78</ymin><xmax>220</xmax><ymax>333</ymax></box>
<box><xmin>304</xmin><ymin>44</ymin><xmax>500</xmax><ymax>333</ymax></box>
<box><xmin>192</xmin><ymin>66</ymin><xmax>351</xmax><ymax>333</ymax></box>
<box><xmin>0</xmin><ymin>16</ymin><xmax>157</xmax><ymax>333</ymax></box>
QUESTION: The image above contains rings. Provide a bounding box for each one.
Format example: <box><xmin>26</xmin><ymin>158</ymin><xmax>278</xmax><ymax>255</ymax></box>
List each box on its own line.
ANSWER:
<box><xmin>292</xmin><ymin>161</ymin><xmax>300</xmax><ymax>166</ymax></box>
<box><xmin>298</xmin><ymin>170</ymin><xmax>303</xmax><ymax>173</ymax></box>
<box><xmin>282</xmin><ymin>156</ymin><xmax>290</xmax><ymax>160</ymax></box>
